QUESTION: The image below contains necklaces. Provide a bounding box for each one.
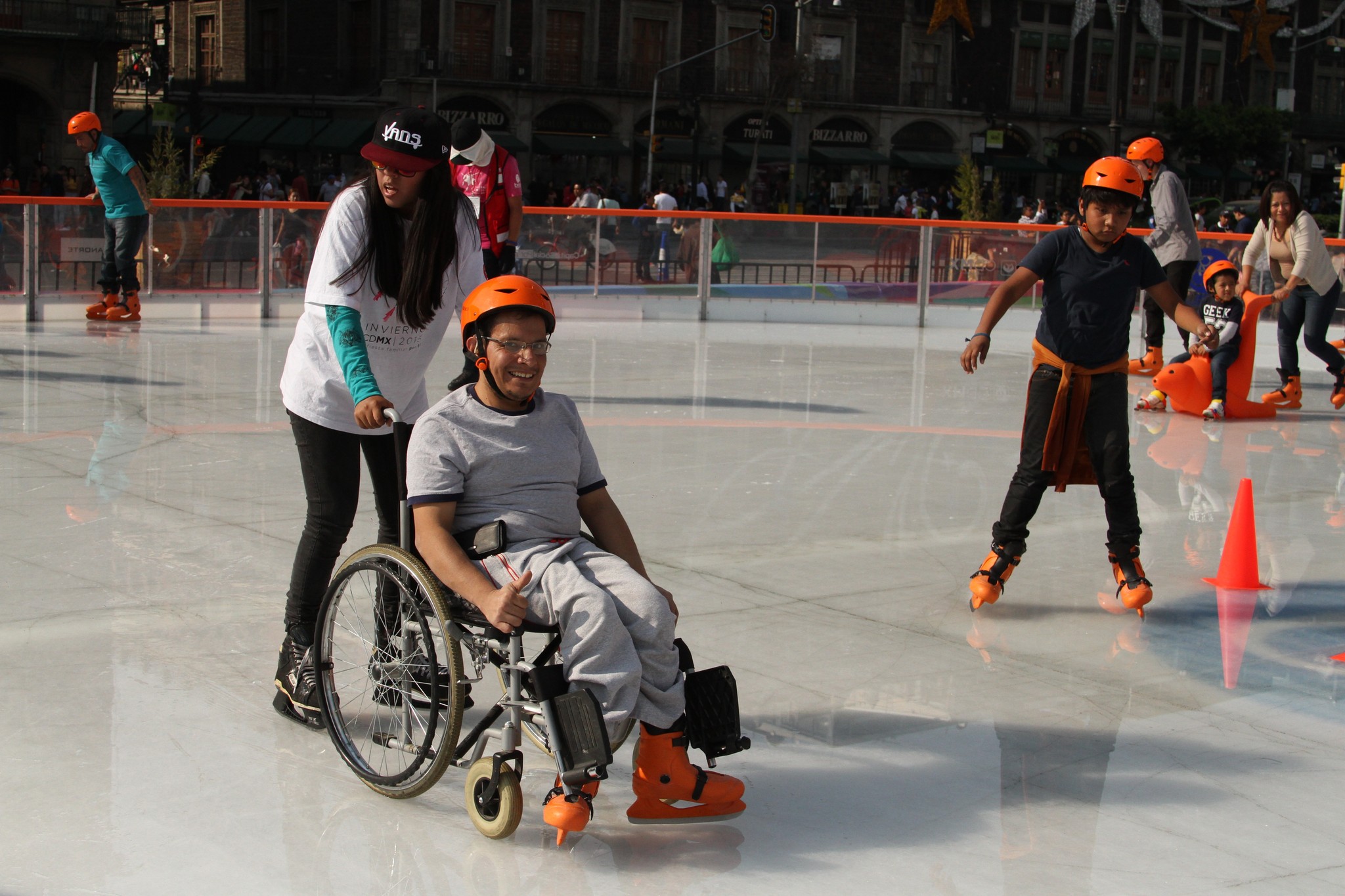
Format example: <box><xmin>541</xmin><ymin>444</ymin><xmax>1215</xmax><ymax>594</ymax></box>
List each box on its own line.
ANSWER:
<box><xmin>1273</xmin><ymin>220</ymin><xmax>1287</xmax><ymax>242</ymax></box>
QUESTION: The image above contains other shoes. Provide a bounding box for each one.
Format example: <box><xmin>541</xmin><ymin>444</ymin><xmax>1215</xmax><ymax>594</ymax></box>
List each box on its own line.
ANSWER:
<box><xmin>447</xmin><ymin>370</ymin><xmax>479</xmax><ymax>391</ymax></box>
<box><xmin>1201</xmin><ymin>422</ymin><xmax>1223</xmax><ymax>442</ymax></box>
<box><xmin>1134</xmin><ymin>391</ymin><xmax>1167</xmax><ymax>412</ymax></box>
<box><xmin>1202</xmin><ymin>400</ymin><xmax>1225</xmax><ymax>421</ymax></box>
<box><xmin>1136</xmin><ymin>413</ymin><xmax>1166</xmax><ymax>435</ymax></box>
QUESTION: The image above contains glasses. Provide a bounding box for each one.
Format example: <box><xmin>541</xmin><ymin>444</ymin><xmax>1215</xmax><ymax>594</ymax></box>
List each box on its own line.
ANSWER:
<box><xmin>474</xmin><ymin>336</ymin><xmax>551</xmax><ymax>356</ymax></box>
<box><xmin>370</xmin><ymin>160</ymin><xmax>416</xmax><ymax>178</ymax></box>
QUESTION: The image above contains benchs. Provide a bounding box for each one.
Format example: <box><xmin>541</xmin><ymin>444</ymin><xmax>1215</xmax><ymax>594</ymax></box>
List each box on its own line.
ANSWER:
<box><xmin>158</xmin><ymin>237</ymin><xmax>291</xmax><ymax>289</ymax></box>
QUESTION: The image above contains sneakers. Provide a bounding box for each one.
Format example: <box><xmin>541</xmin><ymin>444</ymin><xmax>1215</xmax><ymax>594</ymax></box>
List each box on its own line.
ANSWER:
<box><xmin>1272</xmin><ymin>413</ymin><xmax>1301</xmax><ymax>438</ymax></box>
<box><xmin>1128</xmin><ymin>337</ymin><xmax>1164</xmax><ymax>376</ymax></box>
<box><xmin>968</xmin><ymin>537</ymin><xmax>1028</xmax><ymax>613</ymax></box>
<box><xmin>368</xmin><ymin>606</ymin><xmax>474</xmax><ymax>712</ymax></box>
<box><xmin>1330</xmin><ymin>417</ymin><xmax>1345</xmax><ymax>437</ymax></box>
<box><xmin>543</xmin><ymin>772</ymin><xmax>600</xmax><ymax>850</ymax></box>
<box><xmin>273</xmin><ymin>626</ymin><xmax>340</xmax><ymax>732</ymax></box>
<box><xmin>1103</xmin><ymin>542</ymin><xmax>1154</xmax><ymax>622</ymax></box>
<box><xmin>1326</xmin><ymin>364</ymin><xmax>1345</xmax><ymax>410</ymax></box>
<box><xmin>86</xmin><ymin>321</ymin><xmax>106</xmax><ymax>339</ymax></box>
<box><xmin>1261</xmin><ymin>367</ymin><xmax>1303</xmax><ymax>410</ymax></box>
<box><xmin>106</xmin><ymin>290</ymin><xmax>141</xmax><ymax>321</ymax></box>
<box><xmin>626</xmin><ymin>721</ymin><xmax>748</xmax><ymax>824</ymax></box>
<box><xmin>86</xmin><ymin>289</ymin><xmax>119</xmax><ymax>320</ymax></box>
<box><xmin>105</xmin><ymin>322</ymin><xmax>141</xmax><ymax>344</ymax></box>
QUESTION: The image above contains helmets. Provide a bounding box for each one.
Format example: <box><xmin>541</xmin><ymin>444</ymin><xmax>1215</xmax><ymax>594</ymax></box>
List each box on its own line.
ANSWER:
<box><xmin>1126</xmin><ymin>137</ymin><xmax>1164</xmax><ymax>162</ymax></box>
<box><xmin>461</xmin><ymin>274</ymin><xmax>556</xmax><ymax>352</ymax></box>
<box><xmin>1203</xmin><ymin>260</ymin><xmax>1239</xmax><ymax>294</ymax></box>
<box><xmin>1082</xmin><ymin>156</ymin><xmax>1144</xmax><ymax>199</ymax></box>
<box><xmin>1183</xmin><ymin>532</ymin><xmax>1214</xmax><ymax>571</ymax></box>
<box><xmin>68</xmin><ymin>111</ymin><xmax>102</xmax><ymax>134</ymax></box>
<box><xmin>1325</xmin><ymin>505</ymin><xmax>1345</xmax><ymax>528</ymax></box>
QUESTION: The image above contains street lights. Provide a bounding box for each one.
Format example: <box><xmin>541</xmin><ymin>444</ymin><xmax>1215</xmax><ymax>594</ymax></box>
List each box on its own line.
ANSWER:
<box><xmin>787</xmin><ymin>0</ymin><xmax>842</xmax><ymax>213</ymax></box>
<box><xmin>1282</xmin><ymin>0</ymin><xmax>1341</xmax><ymax>182</ymax></box>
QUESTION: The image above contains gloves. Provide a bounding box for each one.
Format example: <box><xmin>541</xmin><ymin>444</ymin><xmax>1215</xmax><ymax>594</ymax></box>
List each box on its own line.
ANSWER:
<box><xmin>500</xmin><ymin>245</ymin><xmax>515</xmax><ymax>273</ymax></box>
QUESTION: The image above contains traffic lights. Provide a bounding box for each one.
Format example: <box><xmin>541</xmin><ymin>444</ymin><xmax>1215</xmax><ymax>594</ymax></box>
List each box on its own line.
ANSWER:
<box><xmin>650</xmin><ymin>135</ymin><xmax>665</xmax><ymax>153</ymax></box>
<box><xmin>759</xmin><ymin>3</ymin><xmax>777</xmax><ymax>42</ymax></box>
<box><xmin>192</xmin><ymin>135</ymin><xmax>205</xmax><ymax>155</ymax></box>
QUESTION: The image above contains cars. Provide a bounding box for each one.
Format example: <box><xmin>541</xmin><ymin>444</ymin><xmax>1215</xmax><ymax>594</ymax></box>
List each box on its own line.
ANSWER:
<box><xmin>1186</xmin><ymin>197</ymin><xmax>1261</xmax><ymax>233</ymax></box>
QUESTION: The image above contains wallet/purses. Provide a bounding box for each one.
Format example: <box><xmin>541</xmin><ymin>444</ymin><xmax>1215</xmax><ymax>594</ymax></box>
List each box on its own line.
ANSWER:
<box><xmin>453</xmin><ymin>520</ymin><xmax>507</xmax><ymax>559</ymax></box>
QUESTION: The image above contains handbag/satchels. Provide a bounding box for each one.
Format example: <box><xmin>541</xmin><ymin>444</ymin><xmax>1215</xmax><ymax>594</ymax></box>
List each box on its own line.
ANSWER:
<box><xmin>711</xmin><ymin>221</ymin><xmax>739</xmax><ymax>271</ymax></box>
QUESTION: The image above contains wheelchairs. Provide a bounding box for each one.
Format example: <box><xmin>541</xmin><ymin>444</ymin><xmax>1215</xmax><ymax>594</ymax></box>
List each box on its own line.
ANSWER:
<box><xmin>313</xmin><ymin>375</ymin><xmax>752</xmax><ymax>840</ymax></box>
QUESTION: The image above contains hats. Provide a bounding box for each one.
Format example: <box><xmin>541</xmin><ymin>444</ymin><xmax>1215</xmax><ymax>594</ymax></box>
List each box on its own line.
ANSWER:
<box><xmin>361</xmin><ymin>105</ymin><xmax>452</xmax><ymax>171</ymax></box>
<box><xmin>450</xmin><ymin>118</ymin><xmax>482</xmax><ymax>165</ymax></box>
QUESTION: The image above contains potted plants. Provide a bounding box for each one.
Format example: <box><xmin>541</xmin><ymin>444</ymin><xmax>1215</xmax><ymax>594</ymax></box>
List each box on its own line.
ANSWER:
<box><xmin>138</xmin><ymin>123</ymin><xmax>224</xmax><ymax>288</ymax></box>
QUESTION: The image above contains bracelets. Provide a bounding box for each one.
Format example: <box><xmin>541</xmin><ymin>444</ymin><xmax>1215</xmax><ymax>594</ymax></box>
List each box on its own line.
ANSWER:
<box><xmin>505</xmin><ymin>240</ymin><xmax>518</xmax><ymax>246</ymax></box>
<box><xmin>963</xmin><ymin>333</ymin><xmax>991</xmax><ymax>343</ymax></box>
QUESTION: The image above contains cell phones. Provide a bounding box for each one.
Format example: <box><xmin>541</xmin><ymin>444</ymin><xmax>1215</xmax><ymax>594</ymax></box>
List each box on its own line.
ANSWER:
<box><xmin>1037</xmin><ymin>198</ymin><xmax>1042</xmax><ymax>204</ymax></box>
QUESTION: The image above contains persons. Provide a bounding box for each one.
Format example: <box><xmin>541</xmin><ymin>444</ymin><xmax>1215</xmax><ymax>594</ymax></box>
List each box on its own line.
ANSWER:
<box><xmin>520</xmin><ymin>173</ymin><xmax>1085</xmax><ymax>286</ymax></box>
<box><xmin>67</xmin><ymin>112</ymin><xmax>159</xmax><ymax>322</ymax></box>
<box><xmin>276</xmin><ymin>109</ymin><xmax>490</xmax><ymax>729</ymax></box>
<box><xmin>1133</xmin><ymin>259</ymin><xmax>1245</xmax><ymax>422</ymax></box>
<box><xmin>958</xmin><ymin>156</ymin><xmax>1219</xmax><ymax>620</ymax></box>
<box><xmin>1235</xmin><ymin>179</ymin><xmax>1345</xmax><ymax>410</ymax></box>
<box><xmin>1192</xmin><ymin>203</ymin><xmax>1254</xmax><ymax>284</ymax></box>
<box><xmin>446</xmin><ymin>119</ymin><xmax>523</xmax><ymax>393</ymax></box>
<box><xmin>0</xmin><ymin>157</ymin><xmax>374</xmax><ymax>292</ymax></box>
<box><xmin>1126</xmin><ymin>136</ymin><xmax>1200</xmax><ymax>377</ymax></box>
<box><xmin>403</xmin><ymin>275</ymin><xmax>745</xmax><ymax>844</ymax></box>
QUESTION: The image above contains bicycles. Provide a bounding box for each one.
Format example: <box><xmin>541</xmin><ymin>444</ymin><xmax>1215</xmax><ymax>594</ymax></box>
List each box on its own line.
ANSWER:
<box><xmin>535</xmin><ymin>214</ymin><xmax>617</xmax><ymax>270</ymax></box>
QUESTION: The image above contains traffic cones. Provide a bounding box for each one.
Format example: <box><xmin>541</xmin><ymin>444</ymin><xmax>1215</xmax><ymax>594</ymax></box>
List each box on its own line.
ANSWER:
<box><xmin>1215</xmin><ymin>585</ymin><xmax>1259</xmax><ymax>691</ymax></box>
<box><xmin>1200</xmin><ymin>476</ymin><xmax>1275</xmax><ymax>591</ymax></box>
<box><xmin>654</xmin><ymin>230</ymin><xmax>678</xmax><ymax>283</ymax></box>
